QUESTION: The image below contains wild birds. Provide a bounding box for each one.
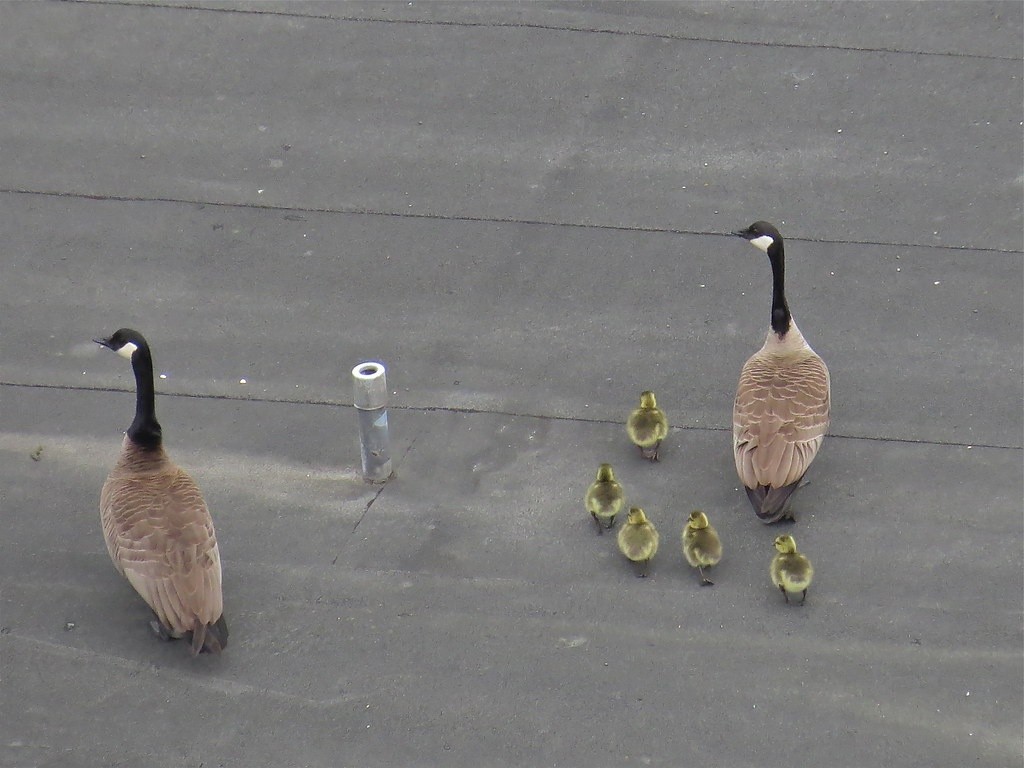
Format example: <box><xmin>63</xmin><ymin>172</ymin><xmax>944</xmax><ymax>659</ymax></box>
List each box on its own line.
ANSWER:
<box><xmin>730</xmin><ymin>221</ymin><xmax>830</xmax><ymax>525</ymax></box>
<box><xmin>627</xmin><ymin>390</ymin><xmax>667</xmax><ymax>460</ymax></box>
<box><xmin>770</xmin><ymin>534</ymin><xmax>814</xmax><ymax>603</ymax></box>
<box><xmin>584</xmin><ymin>463</ymin><xmax>622</xmax><ymax>531</ymax></box>
<box><xmin>682</xmin><ymin>512</ymin><xmax>723</xmax><ymax>585</ymax></box>
<box><xmin>617</xmin><ymin>507</ymin><xmax>659</xmax><ymax>576</ymax></box>
<box><xmin>92</xmin><ymin>329</ymin><xmax>228</xmax><ymax>658</ymax></box>
